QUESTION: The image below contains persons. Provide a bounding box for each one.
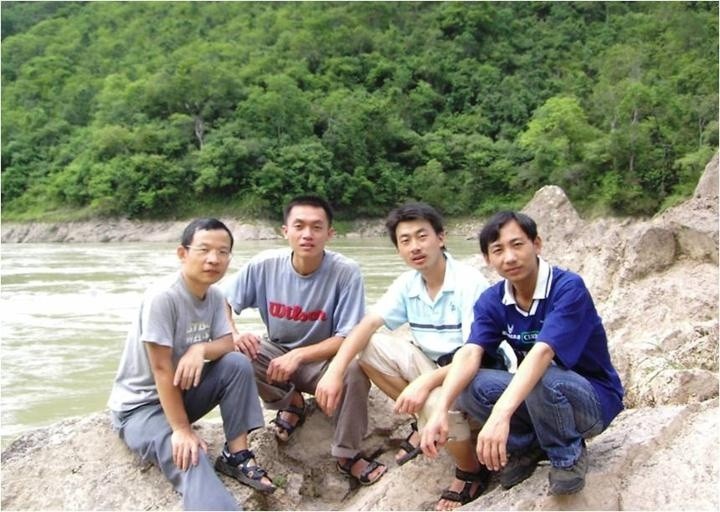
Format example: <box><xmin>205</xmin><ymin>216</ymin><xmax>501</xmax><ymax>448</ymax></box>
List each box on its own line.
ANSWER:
<box><xmin>106</xmin><ymin>218</ymin><xmax>277</xmax><ymax>511</ymax></box>
<box><xmin>314</xmin><ymin>202</ymin><xmax>517</xmax><ymax>511</ymax></box>
<box><xmin>222</xmin><ymin>195</ymin><xmax>387</xmax><ymax>485</ymax></box>
<box><xmin>420</xmin><ymin>211</ymin><xmax>624</xmax><ymax>495</ymax></box>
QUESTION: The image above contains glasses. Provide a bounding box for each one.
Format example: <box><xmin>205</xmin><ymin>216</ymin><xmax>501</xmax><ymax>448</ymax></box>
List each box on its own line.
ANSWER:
<box><xmin>184</xmin><ymin>244</ymin><xmax>233</xmax><ymax>261</ymax></box>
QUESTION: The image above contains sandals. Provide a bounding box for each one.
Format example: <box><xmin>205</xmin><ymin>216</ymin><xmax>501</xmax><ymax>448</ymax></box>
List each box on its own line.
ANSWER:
<box><xmin>337</xmin><ymin>454</ymin><xmax>387</xmax><ymax>486</ymax></box>
<box><xmin>268</xmin><ymin>393</ymin><xmax>307</xmax><ymax>443</ymax></box>
<box><xmin>441</xmin><ymin>467</ymin><xmax>490</xmax><ymax>504</ymax></box>
<box><xmin>214</xmin><ymin>441</ymin><xmax>277</xmax><ymax>493</ymax></box>
<box><xmin>396</xmin><ymin>422</ymin><xmax>422</xmax><ymax>465</ymax></box>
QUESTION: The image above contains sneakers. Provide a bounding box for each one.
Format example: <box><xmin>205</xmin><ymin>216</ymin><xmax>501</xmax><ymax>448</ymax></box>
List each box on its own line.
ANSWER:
<box><xmin>499</xmin><ymin>449</ymin><xmax>548</xmax><ymax>488</ymax></box>
<box><xmin>549</xmin><ymin>441</ymin><xmax>588</xmax><ymax>495</ymax></box>
